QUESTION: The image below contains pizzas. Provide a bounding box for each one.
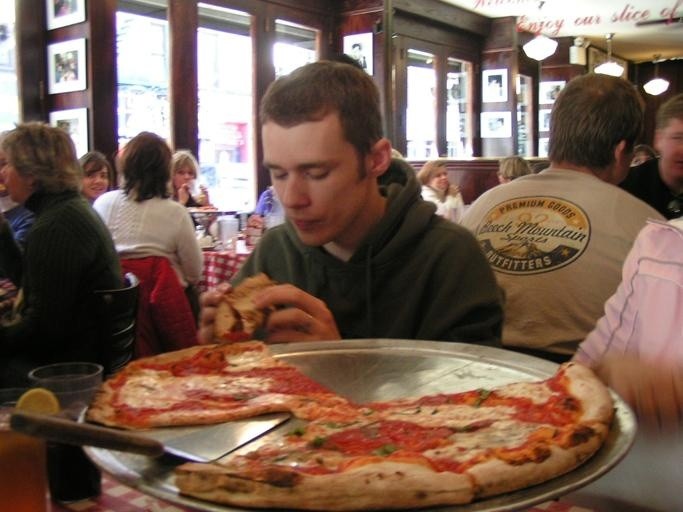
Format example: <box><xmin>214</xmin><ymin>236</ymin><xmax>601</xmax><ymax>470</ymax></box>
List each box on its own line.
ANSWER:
<box><xmin>207</xmin><ymin>271</ymin><xmax>278</xmax><ymax>344</ymax></box>
<box><xmin>85</xmin><ymin>339</ymin><xmax>616</xmax><ymax>511</ymax></box>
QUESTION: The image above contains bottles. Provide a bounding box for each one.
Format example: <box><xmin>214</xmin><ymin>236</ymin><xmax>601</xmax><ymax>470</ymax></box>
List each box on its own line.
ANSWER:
<box><xmin>195</xmin><ymin>225</ymin><xmax>205</xmax><ymax>239</ymax></box>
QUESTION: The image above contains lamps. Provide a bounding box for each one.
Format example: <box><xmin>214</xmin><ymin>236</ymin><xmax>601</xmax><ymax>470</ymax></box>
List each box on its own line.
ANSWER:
<box><xmin>522</xmin><ymin>0</ymin><xmax>561</xmax><ymax>61</ymax></box>
<box><xmin>642</xmin><ymin>58</ymin><xmax>669</xmax><ymax>97</ymax></box>
<box><xmin>593</xmin><ymin>33</ymin><xmax>626</xmax><ymax>78</ymax></box>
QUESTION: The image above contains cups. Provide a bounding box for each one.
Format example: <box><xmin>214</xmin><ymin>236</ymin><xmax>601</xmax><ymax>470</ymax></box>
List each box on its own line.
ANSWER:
<box><xmin>186</xmin><ymin>179</ymin><xmax>205</xmax><ymax>203</ymax></box>
<box><xmin>0</xmin><ymin>387</ymin><xmax>52</xmax><ymax>512</ymax></box>
<box><xmin>449</xmin><ymin>208</ymin><xmax>457</xmax><ymax>224</ymax></box>
<box><xmin>217</xmin><ymin>219</ymin><xmax>239</xmax><ymax>250</ymax></box>
<box><xmin>29</xmin><ymin>361</ymin><xmax>103</xmax><ymax>499</ymax></box>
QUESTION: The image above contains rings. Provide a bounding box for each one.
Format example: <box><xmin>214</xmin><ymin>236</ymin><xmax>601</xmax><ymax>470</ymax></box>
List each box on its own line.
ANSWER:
<box><xmin>294</xmin><ymin>312</ymin><xmax>314</xmax><ymax>334</ymax></box>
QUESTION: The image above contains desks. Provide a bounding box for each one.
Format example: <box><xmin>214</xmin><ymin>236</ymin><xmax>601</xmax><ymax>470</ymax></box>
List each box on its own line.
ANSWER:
<box><xmin>0</xmin><ymin>276</ymin><xmax>17</xmax><ymax>309</ymax></box>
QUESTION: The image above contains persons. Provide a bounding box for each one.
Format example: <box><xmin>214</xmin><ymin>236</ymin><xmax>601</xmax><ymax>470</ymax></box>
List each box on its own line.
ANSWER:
<box><xmin>351</xmin><ymin>43</ymin><xmax>367</xmax><ymax>70</ymax></box>
<box><xmin>196</xmin><ymin>60</ymin><xmax>504</xmax><ymax>347</ymax></box>
<box><xmin>1</xmin><ymin>120</ymin><xmax>213</xmax><ymax>390</ymax></box>
<box><xmin>457</xmin><ymin>73</ymin><xmax>680</xmax><ymax>436</ymax></box>
<box><xmin>410</xmin><ymin>158</ymin><xmax>465</xmax><ymax>222</ymax></box>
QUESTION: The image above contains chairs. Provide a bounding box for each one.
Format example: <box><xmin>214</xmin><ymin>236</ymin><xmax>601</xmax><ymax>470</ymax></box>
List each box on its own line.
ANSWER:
<box><xmin>93</xmin><ymin>273</ymin><xmax>141</xmax><ymax>379</ymax></box>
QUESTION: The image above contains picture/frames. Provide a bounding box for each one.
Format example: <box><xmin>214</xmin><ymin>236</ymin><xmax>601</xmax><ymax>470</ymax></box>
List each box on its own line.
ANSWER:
<box><xmin>341</xmin><ymin>31</ymin><xmax>373</xmax><ymax>77</ymax></box>
<box><xmin>50</xmin><ymin>107</ymin><xmax>88</xmax><ymax>163</ymax></box>
<box><xmin>43</xmin><ymin>0</ymin><xmax>86</xmax><ymax>33</ymax></box>
<box><xmin>479</xmin><ymin>111</ymin><xmax>512</xmax><ymax>139</ymax></box>
<box><xmin>482</xmin><ymin>68</ymin><xmax>509</xmax><ymax>102</ymax></box>
<box><xmin>48</xmin><ymin>37</ymin><xmax>87</xmax><ymax>94</ymax></box>
<box><xmin>537</xmin><ymin>81</ymin><xmax>569</xmax><ymax>157</ymax></box>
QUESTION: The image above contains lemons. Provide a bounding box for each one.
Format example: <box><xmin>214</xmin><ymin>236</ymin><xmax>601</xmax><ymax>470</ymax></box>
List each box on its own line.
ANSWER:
<box><xmin>14</xmin><ymin>387</ymin><xmax>60</xmax><ymax>418</ymax></box>
